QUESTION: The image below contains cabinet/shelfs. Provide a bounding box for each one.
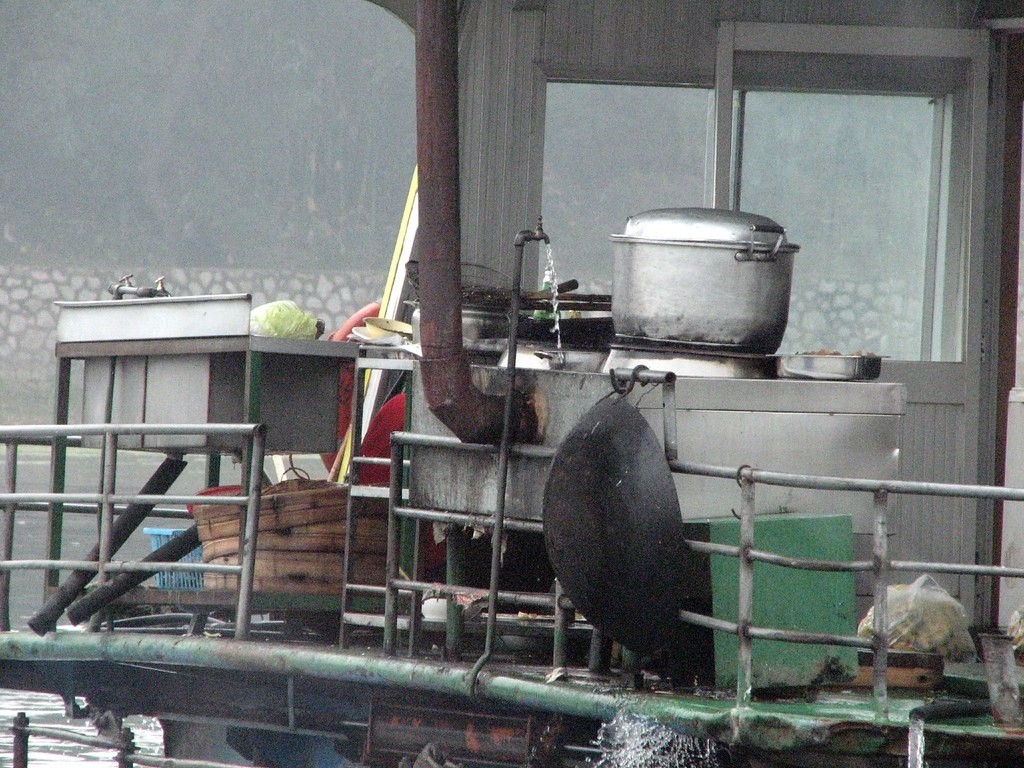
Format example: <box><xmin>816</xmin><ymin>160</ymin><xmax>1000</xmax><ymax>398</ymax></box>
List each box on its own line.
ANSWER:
<box><xmin>338</xmin><ymin>345</ymin><xmax>590</xmax><ymax>663</ymax></box>
<box><xmin>681</xmin><ymin>513</ymin><xmax>859</xmax><ymax>694</ymax></box>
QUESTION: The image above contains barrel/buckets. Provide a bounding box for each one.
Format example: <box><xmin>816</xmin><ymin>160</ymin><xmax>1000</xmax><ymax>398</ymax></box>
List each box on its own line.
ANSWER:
<box><xmin>978</xmin><ymin>634</ymin><xmax>1024</xmax><ymax>728</ymax></box>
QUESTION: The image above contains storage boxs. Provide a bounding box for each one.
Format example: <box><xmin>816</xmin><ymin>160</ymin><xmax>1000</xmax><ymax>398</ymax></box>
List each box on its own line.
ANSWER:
<box><xmin>141</xmin><ymin>528</ymin><xmax>204</xmax><ymax>587</ymax></box>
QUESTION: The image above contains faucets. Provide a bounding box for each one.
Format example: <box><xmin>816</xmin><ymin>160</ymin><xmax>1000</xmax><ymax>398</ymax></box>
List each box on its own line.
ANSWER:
<box><xmin>533</xmin><ymin>214</ymin><xmax>549</xmax><ymax>244</ymax></box>
<box><xmin>120</xmin><ymin>274</ymin><xmax>136</xmax><ymax>299</ymax></box>
<box><xmin>152</xmin><ymin>276</ymin><xmax>171</xmax><ymax>297</ymax></box>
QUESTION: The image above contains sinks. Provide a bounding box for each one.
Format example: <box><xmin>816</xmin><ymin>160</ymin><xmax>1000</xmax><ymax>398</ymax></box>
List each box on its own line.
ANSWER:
<box><xmin>409</xmin><ymin>350</ymin><xmax>906</xmax><ymax>533</ymax></box>
<box><xmin>56</xmin><ymin>336</ymin><xmax>359</xmax><ymax>454</ymax></box>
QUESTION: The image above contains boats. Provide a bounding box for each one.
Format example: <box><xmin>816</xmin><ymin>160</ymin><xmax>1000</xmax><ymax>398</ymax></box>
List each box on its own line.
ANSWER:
<box><xmin>0</xmin><ymin>0</ymin><xmax>1021</xmax><ymax>767</ymax></box>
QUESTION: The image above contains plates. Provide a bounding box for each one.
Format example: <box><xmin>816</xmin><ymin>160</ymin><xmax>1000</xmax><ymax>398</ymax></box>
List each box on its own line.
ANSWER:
<box><xmin>352</xmin><ymin>327</ymin><xmax>397</xmax><ymax>344</ymax></box>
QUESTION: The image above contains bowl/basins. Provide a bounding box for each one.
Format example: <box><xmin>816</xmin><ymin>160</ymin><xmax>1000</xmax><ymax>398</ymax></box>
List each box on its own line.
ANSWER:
<box><xmin>362</xmin><ymin>317</ymin><xmax>412</xmax><ymax>341</ymax></box>
<box><xmin>765</xmin><ymin>353</ymin><xmax>892</xmax><ymax>379</ymax></box>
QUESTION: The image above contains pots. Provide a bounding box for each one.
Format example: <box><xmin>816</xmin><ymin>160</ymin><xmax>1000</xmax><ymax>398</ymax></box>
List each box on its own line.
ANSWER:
<box><xmin>541</xmin><ymin>388</ymin><xmax>707</xmax><ymax>679</ymax></box>
<box><xmin>402</xmin><ymin>298</ymin><xmax>510</xmax><ymax>345</ymax></box>
<box><xmin>607</xmin><ymin>206</ymin><xmax>801</xmax><ymax>349</ymax></box>
